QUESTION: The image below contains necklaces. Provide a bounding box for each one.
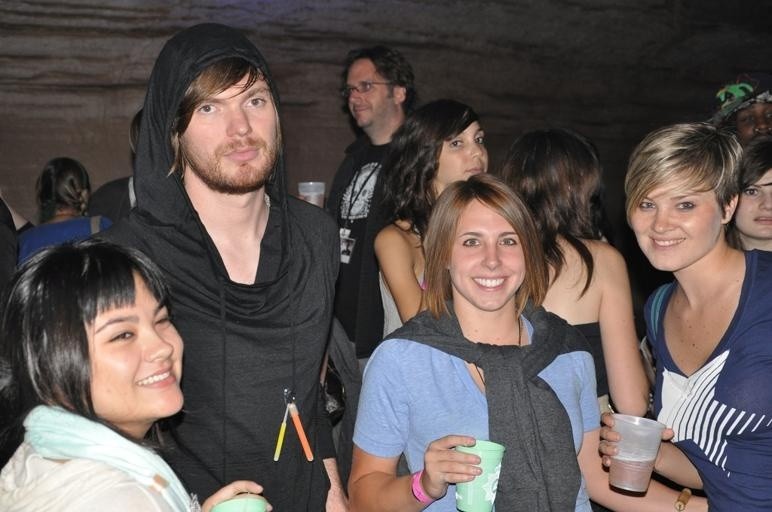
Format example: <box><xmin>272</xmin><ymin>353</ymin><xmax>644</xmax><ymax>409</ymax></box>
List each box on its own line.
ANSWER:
<box><xmin>475</xmin><ymin>319</ymin><xmax>521</xmax><ymax>385</ymax></box>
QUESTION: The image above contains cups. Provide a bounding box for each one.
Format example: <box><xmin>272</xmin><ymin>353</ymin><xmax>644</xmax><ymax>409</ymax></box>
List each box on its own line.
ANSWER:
<box><xmin>608</xmin><ymin>411</ymin><xmax>667</xmax><ymax>495</ymax></box>
<box><xmin>297</xmin><ymin>180</ymin><xmax>327</xmax><ymax>208</ymax></box>
<box><xmin>210</xmin><ymin>493</ymin><xmax>268</xmax><ymax>512</ymax></box>
<box><xmin>454</xmin><ymin>439</ymin><xmax>507</xmax><ymax>512</ymax></box>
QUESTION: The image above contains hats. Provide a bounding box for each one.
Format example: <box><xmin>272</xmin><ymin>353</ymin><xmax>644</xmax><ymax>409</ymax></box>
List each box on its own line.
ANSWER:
<box><xmin>707</xmin><ymin>74</ymin><xmax>770</xmax><ymax>128</ymax></box>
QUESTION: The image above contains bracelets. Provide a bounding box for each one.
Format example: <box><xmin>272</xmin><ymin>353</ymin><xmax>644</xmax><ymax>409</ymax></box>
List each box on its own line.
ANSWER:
<box><xmin>675</xmin><ymin>488</ymin><xmax>692</xmax><ymax>512</ymax></box>
<box><xmin>412</xmin><ymin>470</ymin><xmax>436</xmax><ymax>504</ymax></box>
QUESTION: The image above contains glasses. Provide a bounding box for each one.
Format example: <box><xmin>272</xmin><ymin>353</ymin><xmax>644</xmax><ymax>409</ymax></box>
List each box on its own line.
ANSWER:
<box><xmin>340</xmin><ymin>82</ymin><xmax>389</xmax><ymax>98</ymax></box>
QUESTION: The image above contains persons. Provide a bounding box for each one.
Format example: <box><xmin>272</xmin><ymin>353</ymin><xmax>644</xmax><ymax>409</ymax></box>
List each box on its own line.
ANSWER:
<box><xmin>375</xmin><ymin>102</ymin><xmax>488</xmax><ymax>336</ymax></box>
<box><xmin>2</xmin><ymin>201</ymin><xmax>35</xmax><ymax>294</ymax></box>
<box><xmin>85</xmin><ymin>176</ymin><xmax>138</xmax><ymax>223</ymax></box>
<box><xmin>342</xmin><ymin>241</ymin><xmax>351</xmax><ymax>255</ymax></box>
<box><xmin>495</xmin><ymin>126</ymin><xmax>656</xmax><ymax>418</ymax></box>
<box><xmin>100</xmin><ymin>21</ymin><xmax>350</xmax><ymax>511</ymax></box>
<box><xmin>299</xmin><ymin>46</ymin><xmax>418</xmax><ymax>497</ymax></box>
<box><xmin>18</xmin><ymin>156</ymin><xmax>113</xmax><ymax>259</ymax></box>
<box><xmin>350</xmin><ymin>172</ymin><xmax>709</xmax><ymax>511</ymax></box>
<box><xmin>712</xmin><ymin>74</ymin><xmax>772</xmax><ymax>145</ymax></box>
<box><xmin>3</xmin><ymin>237</ymin><xmax>274</xmax><ymax>511</ymax></box>
<box><xmin>726</xmin><ymin>136</ymin><xmax>772</xmax><ymax>253</ymax></box>
<box><xmin>599</xmin><ymin>123</ymin><xmax>772</xmax><ymax>512</ymax></box>
